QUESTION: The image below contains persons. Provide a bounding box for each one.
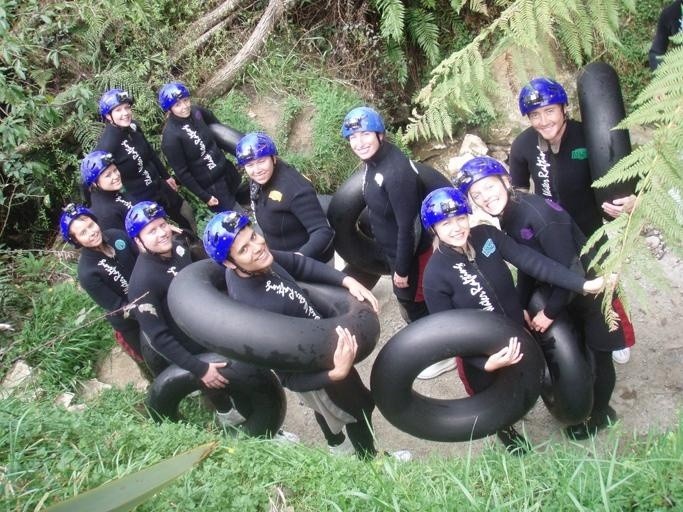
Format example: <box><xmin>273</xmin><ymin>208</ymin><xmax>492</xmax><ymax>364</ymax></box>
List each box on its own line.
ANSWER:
<box><xmin>340</xmin><ymin>106</ymin><xmax>458</xmax><ymax>379</ymax></box>
<box><xmin>421</xmin><ymin>187</ymin><xmax>617</xmax><ymax>456</ymax></box>
<box><xmin>456</xmin><ymin>157</ymin><xmax>635</xmax><ymax>441</ymax></box>
<box><xmin>60</xmin><ymin>206</ymin><xmax>155</xmax><ymax>384</ymax></box>
<box><xmin>125</xmin><ymin>201</ymin><xmax>299</xmax><ymax>443</ymax></box>
<box><xmin>509</xmin><ymin>77</ymin><xmax>638</xmax><ymax>364</ymax></box>
<box><xmin>79</xmin><ymin>151</ymin><xmax>185</xmax><ymax>245</ymax></box>
<box><xmin>235</xmin><ymin>133</ymin><xmax>336</xmax><ymax>268</ymax></box>
<box><xmin>159</xmin><ymin>82</ymin><xmax>250</xmax><ymax>214</ymax></box>
<box><xmin>95</xmin><ymin>89</ymin><xmax>197</xmax><ymax>236</ymax></box>
<box><xmin>203</xmin><ymin>210</ymin><xmax>412</xmax><ymax>465</ymax></box>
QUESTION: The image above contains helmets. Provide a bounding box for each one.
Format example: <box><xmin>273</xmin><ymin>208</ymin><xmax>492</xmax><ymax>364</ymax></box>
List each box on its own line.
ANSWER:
<box><xmin>420</xmin><ymin>186</ymin><xmax>473</xmax><ymax>236</ymax></box>
<box><xmin>519</xmin><ymin>77</ymin><xmax>568</xmax><ymax>116</ymax></box>
<box><xmin>158</xmin><ymin>80</ymin><xmax>191</xmax><ymax>112</ymax></box>
<box><xmin>59</xmin><ymin>205</ymin><xmax>98</xmax><ymax>243</ymax></box>
<box><xmin>341</xmin><ymin>106</ymin><xmax>386</xmax><ymax>140</ymax></box>
<box><xmin>80</xmin><ymin>149</ymin><xmax>116</xmax><ymax>186</ymax></box>
<box><xmin>458</xmin><ymin>156</ymin><xmax>509</xmax><ymax>192</ymax></box>
<box><xmin>124</xmin><ymin>200</ymin><xmax>167</xmax><ymax>240</ymax></box>
<box><xmin>99</xmin><ymin>89</ymin><xmax>133</xmax><ymax>116</ymax></box>
<box><xmin>202</xmin><ymin>209</ymin><xmax>252</xmax><ymax>267</ymax></box>
<box><xmin>235</xmin><ymin>131</ymin><xmax>278</xmax><ymax>166</ymax></box>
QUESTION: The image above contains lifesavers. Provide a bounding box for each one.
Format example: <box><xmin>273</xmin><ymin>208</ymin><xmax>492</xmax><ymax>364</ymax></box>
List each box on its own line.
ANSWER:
<box><xmin>167</xmin><ymin>258</ymin><xmax>379</xmax><ymax>371</ymax></box>
<box><xmin>578</xmin><ymin>62</ymin><xmax>638</xmax><ymax>222</ymax></box>
<box><xmin>370</xmin><ymin>308</ymin><xmax>545</xmax><ymax>442</ymax></box>
<box><xmin>328</xmin><ymin>162</ymin><xmax>453</xmax><ymax>276</ymax></box>
<box><xmin>146</xmin><ymin>352</ymin><xmax>286</xmax><ymax>440</ymax></box>
<box><xmin>528</xmin><ymin>281</ymin><xmax>594</xmax><ymax>424</ymax></box>
<box><xmin>250</xmin><ymin>195</ymin><xmax>381</xmax><ymax>291</ymax></box>
<box><xmin>207</xmin><ymin>122</ymin><xmax>250</xmax><ymax>206</ymax></box>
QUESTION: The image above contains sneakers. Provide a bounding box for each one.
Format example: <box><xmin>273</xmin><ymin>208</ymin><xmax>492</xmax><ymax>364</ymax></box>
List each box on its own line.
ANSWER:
<box><xmin>357</xmin><ymin>450</ymin><xmax>411</xmax><ymax>475</ymax></box>
<box><xmin>328</xmin><ymin>435</ymin><xmax>355</xmax><ymax>456</ymax></box>
<box><xmin>217</xmin><ymin>408</ymin><xmax>247</xmax><ymax>427</ymax></box>
<box><xmin>566</xmin><ymin>405</ymin><xmax>608</xmax><ymax>439</ymax></box>
<box><xmin>606</xmin><ymin>403</ymin><xmax>617</xmax><ymax>426</ymax></box>
<box><xmin>417</xmin><ymin>357</ymin><xmax>458</xmax><ymax>379</ymax></box>
<box><xmin>612</xmin><ymin>345</ymin><xmax>630</xmax><ymax>364</ymax></box>
<box><xmin>497</xmin><ymin>425</ymin><xmax>531</xmax><ymax>456</ymax></box>
<box><xmin>271</xmin><ymin>429</ymin><xmax>299</xmax><ymax>446</ymax></box>
